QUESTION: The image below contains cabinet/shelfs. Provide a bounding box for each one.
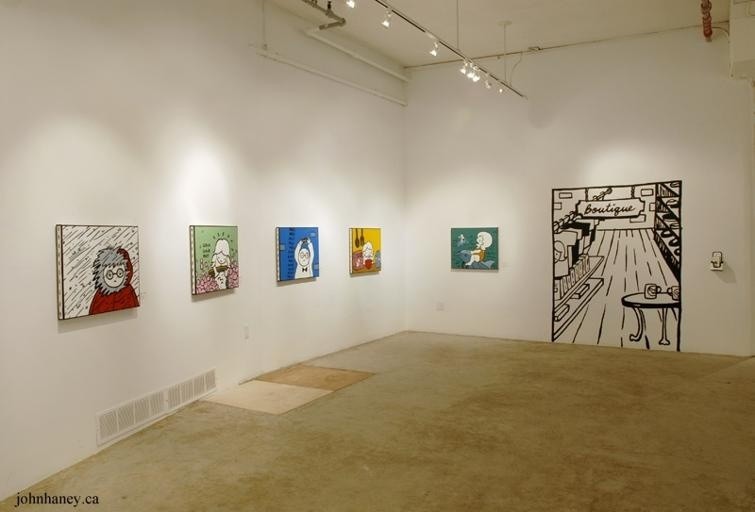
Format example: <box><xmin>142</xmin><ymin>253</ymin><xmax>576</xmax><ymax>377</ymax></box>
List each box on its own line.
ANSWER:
<box><xmin>654</xmin><ymin>182</ymin><xmax>681</xmax><ymax>281</ymax></box>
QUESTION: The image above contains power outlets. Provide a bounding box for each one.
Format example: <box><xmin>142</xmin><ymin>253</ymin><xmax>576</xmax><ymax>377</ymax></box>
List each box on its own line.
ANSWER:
<box><xmin>710</xmin><ymin>258</ymin><xmax>723</xmax><ymax>270</ymax></box>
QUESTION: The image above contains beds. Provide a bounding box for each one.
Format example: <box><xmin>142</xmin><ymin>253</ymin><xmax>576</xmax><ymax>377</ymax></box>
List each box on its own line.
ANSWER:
<box><xmin>621</xmin><ymin>292</ymin><xmax>680</xmax><ymax>346</ymax></box>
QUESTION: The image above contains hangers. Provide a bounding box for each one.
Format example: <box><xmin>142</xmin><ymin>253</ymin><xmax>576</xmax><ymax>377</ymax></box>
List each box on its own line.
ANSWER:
<box><xmin>553</xmin><ymin>211</ymin><xmax>576</xmax><ymax>233</ymax></box>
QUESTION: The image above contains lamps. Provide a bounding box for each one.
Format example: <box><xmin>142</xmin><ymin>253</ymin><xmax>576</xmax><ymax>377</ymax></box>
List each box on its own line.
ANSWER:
<box><xmin>376</xmin><ymin>0</ymin><xmax>527</xmax><ymax>100</ymax></box>
